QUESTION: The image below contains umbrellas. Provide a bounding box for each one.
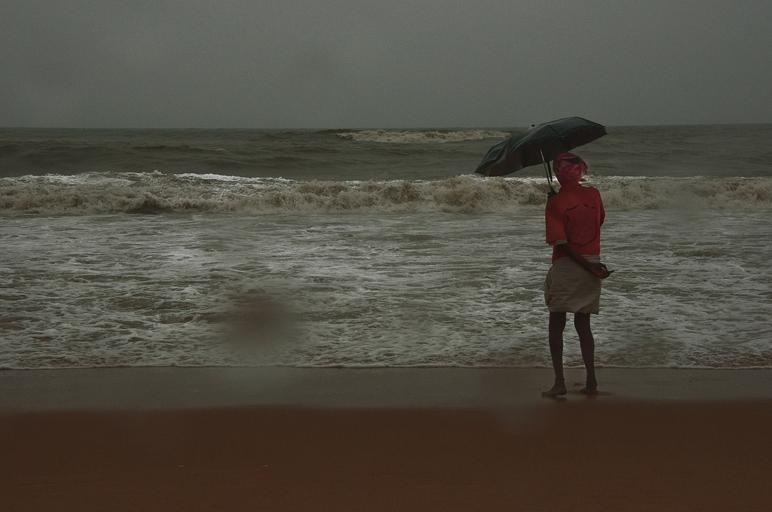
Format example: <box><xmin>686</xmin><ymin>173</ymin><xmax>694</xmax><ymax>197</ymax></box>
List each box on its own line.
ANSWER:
<box><xmin>472</xmin><ymin>115</ymin><xmax>609</xmax><ymax>195</ymax></box>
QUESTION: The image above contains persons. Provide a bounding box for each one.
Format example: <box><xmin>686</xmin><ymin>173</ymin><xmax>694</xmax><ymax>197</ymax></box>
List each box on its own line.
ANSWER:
<box><xmin>538</xmin><ymin>150</ymin><xmax>610</xmax><ymax>399</ymax></box>
